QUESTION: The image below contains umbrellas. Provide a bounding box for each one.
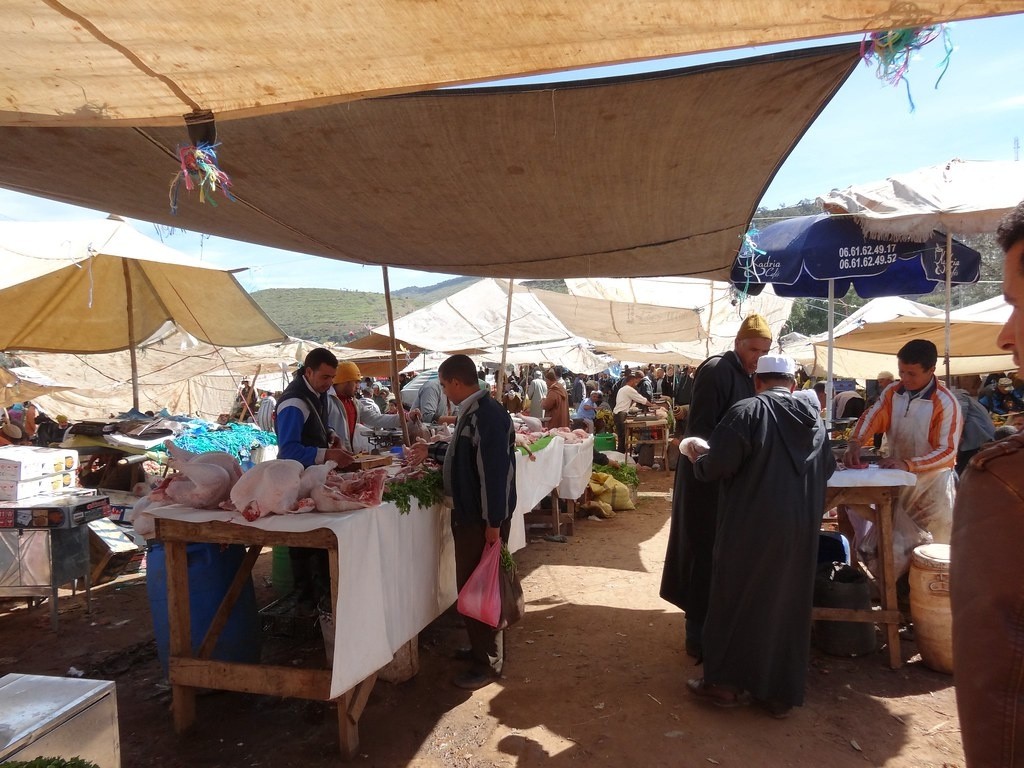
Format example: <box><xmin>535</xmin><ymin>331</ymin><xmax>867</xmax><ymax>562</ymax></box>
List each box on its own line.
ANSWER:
<box><xmin>729</xmin><ymin>213</ymin><xmax>981</xmax><ymax>441</ymax></box>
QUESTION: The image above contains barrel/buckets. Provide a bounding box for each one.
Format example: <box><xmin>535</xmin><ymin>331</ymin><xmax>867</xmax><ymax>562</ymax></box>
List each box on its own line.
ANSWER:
<box><xmin>272</xmin><ymin>546</ymin><xmax>294</xmax><ymax>593</ymax></box>
<box><xmin>146</xmin><ymin>538</ymin><xmax>262</xmax><ymax>683</ymax></box>
<box><xmin>316</xmin><ymin>592</ymin><xmax>419</xmax><ymax>683</ymax></box>
<box><xmin>594</xmin><ymin>433</ymin><xmax>616</xmax><ymax>452</ymax></box>
<box><xmin>909</xmin><ymin>544</ymin><xmax>954</xmax><ymax>674</ymax></box>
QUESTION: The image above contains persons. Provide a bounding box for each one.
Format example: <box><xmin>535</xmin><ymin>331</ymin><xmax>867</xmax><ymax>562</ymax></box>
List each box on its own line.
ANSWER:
<box><xmin>439</xmin><ymin>354</ymin><xmax>519</xmax><ymax>690</ymax></box>
<box><xmin>648</xmin><ymin>363</ymin><xmax>697</xmax><ymax>444</ymax></box>
<box><xmin>326</xmin><ymin>361</ymin><xmax>423</xmax><ymax>471</ymax></box>
<box><xmin>239</xmin><ymin>380</ymin><xmax>258</xmax><ymax>417</ymax></box>
<box><xmin>257</xmin><ymin>391</ymin><xmax>276</xmax><ymax>432</ymax></box>
<box><xmin>952</xmin><ymin>371</ymin><xmax>1024</xmax><ymax>482</ymax></box>
<box><xmin>48</xmin><ymin>415</ymin><xmax>73</xmax><ymax>442</ymax></box>
<box><xmin>410</xmin><ymin>376</ymin><xmax>459</xmax><ymax>426</ymax></box>
<box><xmin>659</xmin><ymin>312</ymin><xmax>836</xmax><ymax>720</ymax></box>
<box><xmin>842</xmin><ymin>338</ymin><xmax>964</xmax><ymax>641</ymax></box>
<box><xmin>520</xmin><ymin>362</ymin><xmax>653</xmax><ymax>472</ymax></box>
<box><xmin>950</xmin><ymin>200</ymin><xmax>1024</xmax><ymax>768</ymax></box>
<box><xmin>8</xmin><ymin>403</ymin><xmax>23</xmax><ymax>423</ymax></box>
<box><xmin>362</xmin><ymin>371</ymin><xmax>419</xmax><ymax>423</ymax></box>
<box><xmin>792</xmin><ymin>370</ymin><xmax>894</xmax><ymax>448</ymax></box>
<box><xmin>275</xmin><ymin>348</ymin><xmax>355</xmax><ymax>609</ymax></box>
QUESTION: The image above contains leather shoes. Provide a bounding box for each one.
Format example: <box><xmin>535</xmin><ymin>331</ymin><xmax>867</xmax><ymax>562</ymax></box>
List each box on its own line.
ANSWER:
<box><xmin>766</xmin><ymin>699</ymin><xmax>792</xmax><ymax>719</ymax></box>
<box><xmin>686</xmin><ymin>676</ymin><xmax>739</xmax><ymax>708</ymax></box>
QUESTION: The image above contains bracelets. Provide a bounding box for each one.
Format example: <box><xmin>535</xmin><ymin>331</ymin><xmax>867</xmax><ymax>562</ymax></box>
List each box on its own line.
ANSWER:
<box><xmin>405</xmin><ymin>413</ymin><xmax>410</xmax><ymax>420</ymax></box>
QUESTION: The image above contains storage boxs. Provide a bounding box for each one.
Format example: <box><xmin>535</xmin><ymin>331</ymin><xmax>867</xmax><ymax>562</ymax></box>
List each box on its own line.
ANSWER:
<box><xmin>0</xmin><ymin>445</ymin><xmax>82</xmax><ymax>500</ymax></box>
<box><xmin>82</xmin><ymin>517</ymin><xmax>138</xmax><ymax>586</ymax></box>
<box><xmin>834</xmin><ymin>378</ymin><xmax>856</xmax><ymax>392</ymax></box>
<box><xmin>124</xmin><ymin>532</ymin><xmax>144</xmax><ymax>574</ymax></box>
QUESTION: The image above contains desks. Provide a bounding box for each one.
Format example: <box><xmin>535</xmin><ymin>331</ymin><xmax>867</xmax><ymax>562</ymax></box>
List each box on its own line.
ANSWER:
<box><xmin>815</xmin><ymin>464</ymin><xmax>917</xmax><ymax>670</ymax></box>
<box><xmin>624</xmin><ymin>398</ymin><xmax>672</xmax><ymax>477</ymax></box>
<box><xmin>134</xmin><ymin>434</ymin><xmax>594</xmax><ymax>754</ymax></box>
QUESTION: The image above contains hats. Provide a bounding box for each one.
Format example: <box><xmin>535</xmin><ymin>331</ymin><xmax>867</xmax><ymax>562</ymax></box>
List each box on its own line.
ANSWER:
<box><xmin>736</xmin><ymin>314</ymin><xmax>773</xmax><ymax>340</ymax></box>
<box><xmin>534</xmin><ymin>371</ymin><xmax>542</xmax><ymax>378</ymax></box>
<box><xmin>878</xmin><ymin>371</ymin><xmax>894</xmax><ymax>381</ymax></box>
<box><xmin>754</xmin><ymin>354</ymin><xmax>795</xmax><ymax>375</ymax></box>
<box><xmin>999</xmin><ymin>378</ymin><xmax>1014</xmax><ymax>391</ymax></box>
<box><xmin>331</xmin><ymin>362</ymin><xmax>362</xmax><ymax>384</ymax></box>
<box><xmin>545</xmin><ymin>372</ymin><xmax>556</xmax><ymax>381</ymax></box>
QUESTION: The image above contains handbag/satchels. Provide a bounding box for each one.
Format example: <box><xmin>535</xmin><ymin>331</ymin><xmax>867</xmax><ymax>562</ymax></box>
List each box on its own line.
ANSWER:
<box><xmin>456</xmin><ymin>540</ymin><xmax>502</xmax><ymax>628</ymax></box>
<box><xmin>492</xmin><ymin>550</ymin><xmax>526</xmax><ymax>632</ymax></box>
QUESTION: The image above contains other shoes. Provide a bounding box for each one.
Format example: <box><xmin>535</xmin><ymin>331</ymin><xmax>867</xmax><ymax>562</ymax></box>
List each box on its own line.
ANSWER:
<box><xmin>457</xmin><ymin>648</ymin><xmax>485</xmax><ymax>660</ymax></box>
<box><xmin>454</xmin><ymin>665</ymin><xmax>500</xmax><ymax>687</ymax></box>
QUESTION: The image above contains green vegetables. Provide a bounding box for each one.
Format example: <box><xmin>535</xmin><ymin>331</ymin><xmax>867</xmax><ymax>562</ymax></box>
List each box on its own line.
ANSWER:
<box><xmin>592</xmin><ymin>461</ymin><xmax>640</xmax><ymax>487</ymax></box>
<box><xmin>379</xmin><ymin>469</ymin><xmax>445</xmax><ymax>514</ymax></box>
